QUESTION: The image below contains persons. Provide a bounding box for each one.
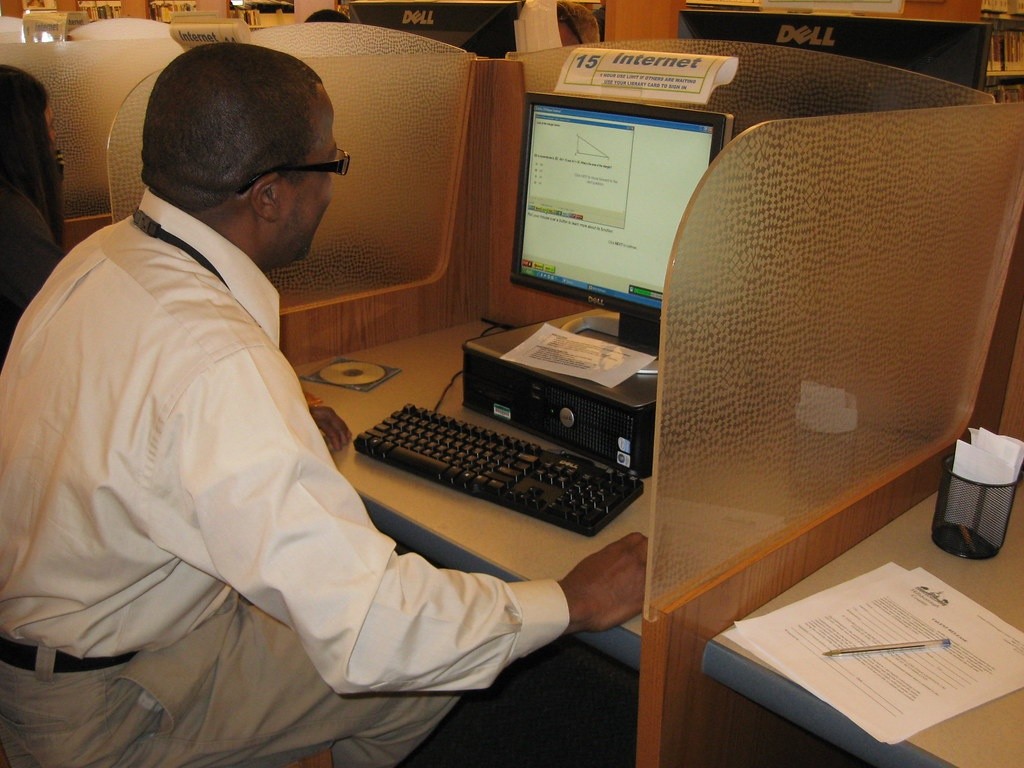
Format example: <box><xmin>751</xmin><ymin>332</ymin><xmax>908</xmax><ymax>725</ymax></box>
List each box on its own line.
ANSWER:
<box><xmin>0</xmin><ymin>43</ymin><xmax>650</xmax><ymax>767</ymax></box>
<box><xmin>0</xmin><ymin>65</ymin><xmax>66</xmax><ymax>373</ymax></box>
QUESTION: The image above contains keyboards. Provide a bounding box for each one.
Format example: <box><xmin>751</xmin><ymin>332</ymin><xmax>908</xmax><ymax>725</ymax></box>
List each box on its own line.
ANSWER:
<box><xmin>354</xmin><ymin>403</ymin><xmax>644</xmax><ymax>537</ymax></box>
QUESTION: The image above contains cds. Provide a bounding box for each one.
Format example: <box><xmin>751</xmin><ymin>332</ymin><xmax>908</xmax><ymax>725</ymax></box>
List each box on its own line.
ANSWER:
<box><xmin>319</xmin><ymin>361</ymin><xmax>385</xmax><ymax>385</ymax></box>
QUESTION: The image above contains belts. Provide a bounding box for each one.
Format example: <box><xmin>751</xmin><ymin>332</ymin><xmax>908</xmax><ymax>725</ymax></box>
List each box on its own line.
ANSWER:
<box><xmin>0</xmin><ymin>639</ymin><xmax>139</xmax><ymax>673</ymax></box>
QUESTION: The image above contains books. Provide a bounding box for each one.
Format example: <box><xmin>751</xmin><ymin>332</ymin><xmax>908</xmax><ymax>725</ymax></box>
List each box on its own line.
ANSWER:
<box><xmin>984</xmin><ymin>30</ymin><xmax>1023</xmax><ymax>72</ymax></box>
<box><xmin>983</xmin><ymin>76</ymin><xmax>1023</xmax><ymax>102</ymax></box>
<box><xmin>979</xmin><ymin>0</ymin><xmax>1024</xmax><ymax>15</ymax></box>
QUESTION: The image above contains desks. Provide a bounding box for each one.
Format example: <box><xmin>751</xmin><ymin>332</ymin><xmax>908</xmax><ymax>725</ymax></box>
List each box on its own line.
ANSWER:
<box><xmin>291</xmin><ymin>315</ymin><xmax>945</xmax><ymax>677</ymax></box>
<box><xmin>700</xmin><ymin>479</ymin><xmax>1022</xmax><ymax>766</ymax></box>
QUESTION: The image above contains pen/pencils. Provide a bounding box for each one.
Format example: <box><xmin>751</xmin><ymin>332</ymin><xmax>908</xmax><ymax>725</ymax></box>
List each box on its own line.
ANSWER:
<box><xmin>820</xmin><ymin>638</ymin><xmax>952</xmax><ymax>659</ymax></box>
<box><xmin>958</xmin><ymin>523</ymin><xmax>977</xmax><ymax>555</ymax></box>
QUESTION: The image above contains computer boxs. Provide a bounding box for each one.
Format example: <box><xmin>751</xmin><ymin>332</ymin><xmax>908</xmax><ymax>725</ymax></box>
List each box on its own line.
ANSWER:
<box><xmin>462</xmin><ymin>309</ymin><xmax>657</xmax><ymax>479</ymax></box>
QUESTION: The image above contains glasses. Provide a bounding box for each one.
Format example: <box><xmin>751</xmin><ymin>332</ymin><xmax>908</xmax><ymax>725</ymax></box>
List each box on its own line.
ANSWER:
<box><xmin>235</xmin><ymin>149</ymin><xmax>351</xmax><ymax>195</ymax></box>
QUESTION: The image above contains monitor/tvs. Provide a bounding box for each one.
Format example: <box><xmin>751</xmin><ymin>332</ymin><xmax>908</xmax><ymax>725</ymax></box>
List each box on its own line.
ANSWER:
<box><xmin>348</xmin><ymin>0</ymin><xmax>523</xmax><ymax>61</ymax></box>
<box><xmin>510</xmin><ymin>91</ymin><xmax>734</xmax><ymax>374</ymax></box>
<box><xmin>675</xmin><ymin>9</ymin><xmax>992</xmax><ymax>92</ymax></box>
